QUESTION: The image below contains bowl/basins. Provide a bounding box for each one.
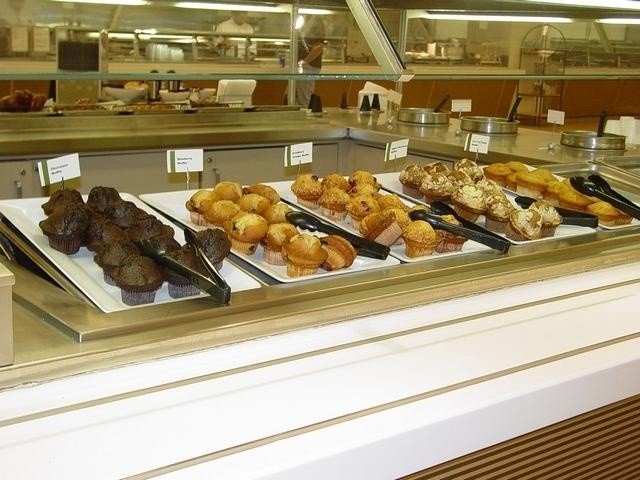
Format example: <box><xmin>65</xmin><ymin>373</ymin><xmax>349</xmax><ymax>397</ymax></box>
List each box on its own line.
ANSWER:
<box><xmin>159</xmin><ymin>89</ymin><xmax>190</xmax><ymax>102</ymax></box>
<box><xmin>102</xmin><ymin>87</ymin><xmax>145</xmax><ymax>102</ymax></box>
<box><xmin>189</xmin><ymin>89</ymin><xmax>216</xmax><ymax>103</ymax></box>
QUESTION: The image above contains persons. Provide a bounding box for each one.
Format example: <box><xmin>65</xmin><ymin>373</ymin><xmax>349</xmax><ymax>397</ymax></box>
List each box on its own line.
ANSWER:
<box><xmin>283</xmin><ymin>6</ymin><xmax>326</xmax><ymax>106</ymax></box>
<box><xmin>213</xmin><ymin>8</ymin><xmax>258</xmax><ymax>107</ymax></box>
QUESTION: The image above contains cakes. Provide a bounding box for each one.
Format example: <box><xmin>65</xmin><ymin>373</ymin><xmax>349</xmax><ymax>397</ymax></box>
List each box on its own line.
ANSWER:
<box><xmin>141</xmin><ymin>235</ymin><xmax>181</xmax><ymax>282</ymax></box>
<box><xmin>505</xmin><ymin>173</ymin><xmax>517</xmax><ymax>191</ymax></box>
<box><xmin>449</xmin><ymin>185</ymin><xmax>487</xmax><ymax>225</ymax></box>
<box><xmin>483</xmin><ymin>162</ymin><xmax>513</xmax><ymax>189</ymax></box>
<box><xmin>290</xmin><ymin>174</ymin><xmax>322</xmax><ymax>209</ymax></box>
<box><xmin>345</xmin><ymin>196</ymin><xmax>381</xmax><ymax>230</ymax></box>
<box><xmin>433</xmin><ymin>214</ymin><xmax>469</xmax><ymax>253</ymax></box>
<box><xmin>617</xmin><ymin>208</ymin><xmax>634</xmax><ymax>226</ymax></box>
<box><xmin>484</xmin><ymin>194</ymin><xmax>516</xmax><ymax>234</ymax></box>
<box><xmin>346</xmin><ymin>183</ymin><xmax>384</xmax><ymax>200</ymax></box>
<box><xmin>559</xmin><ymin>189</ymin><xmax>594</xmax><ymax>212</ymax></box>
<box><xmin>98</xmin><ymin>239</ymin><xmax>142</xmax><ymax>287</ymax></box>
<box><xmin>348</xmin><ymin>171</ymin><xmax>382</xmax><ymax>192</ymax></box>
<box><xmin>399</xmin><ymin>163</ymin><xmax>429</xmax><ymax>199</ymax></box>
<box><xmin>543</xmin><ymin>180</ymin><xmax>569</xmax><ymax>206</ymax></box>
<box><xmin>235</xmin><ymin>193</ymin><xmax>270</xmax><ymax>215</ymax></box>
<box><xmin>529</xmin><ymin>200</ymin><xmax>563</xmax><ymax>238</ymax></box>
<box><xmin>529</xmin><ymin>167</ymin><xmax>560</xmax><ymax>185</ymax></box>
<box><xmin>384</xmin><ymin>208</ymin><xmax>413</xmax><ymax>245</ymax></box>
<box><xmin>419</xmin><ymin>172</ymin><xmax>455</xmax><ymax>205</ymax></box>
<box><xmin>203</xmin><ymin>200</ymin><xmax>241</xmax><ymax>231</ymax></box>
<box><xmin>318</xmin><ymin>188</ymin><xmax>350</xmax><ymax>222</ymax></box>
<box><xmin>358</xmin><ymin>211</ymin><xmax>404</xmax><ymax>251</ymax></box>
<box><xmin>106</xmin><ymin>200</ymin><xmax>147</xmax><ymax>229</ymax></box>
<box><xmin>125</xmin><ymin>213</ymin><xmax>175</xmax><ymax>249</ymax></box>
<box><xmin>261</xmin><ymin>203</ymin><xmax>297</xmax><ymax>248</ymax></box>
<box><xmin>162</xmin><ymin>250</ymin><xmax>209</xmax><ymax>299</ymax></box>
<box><xmin>446</xmin><ymin>169</ymin><xmax>474</xmax><ymax>205</ymax></box>
<box><xmin>281</xmin><ymin>233</ymin><xmax>328</xmax><ymax>278</ymax></box>
<box><xmin>505</xmin><ymin>162</ymin><xmax>528</xmax><ymax>172</ymax></box>
<box><xmin>505</xmin><ymin>208</ymin><xmax>543</xmax><ymax>241</ymax></box>
<box><xmin>400</xmin><ymin>220</ymin><xmax>442</xmax><ymax>259</ymax></box>
<box><xmin>39</xmin><ymin>209</ymin><xmax>88</xmax><ymax>254</ymax></box>
<box><xmin>377</xmin><ymin>194</ymin><xmax>409</xmax><ymax>212</ymax></box>
<box><xmin>243</xmin><ymin>184</ymin><xmax>281</xmax><ymax>205</ymax></box>
<box><xmin>321</xmin><ymin>173</ymin><xmax>351</xmax><ymax>192</ymax></box>
<box><xmin>475</xmin><ymin>179</ymin><xmax>506</xmax><ymax>215</ymax></box>
<box><xmin>424</xmin><ymin>161</ymin><xmax>449</xmax><ymax>177</ymax></box>
<box><xmin>183</xmin><ymin>227</ymin><xmax>231</xmax><ymax>273</ymax></box>
<box><xmin>260</xmin><ymin>222</ymin><xmax>300</xmax><ymax>267</ymax></box>
<box><xmin>586</xmin><ymin>201</ymin><xmax>617</xmax><ymax>227</ymax></box>
<box><xmin>212</xmin><ymin>181</ymin><xmax>243</xmax><ymax>202</ymax></box>
<box><xmin>454</xmin><ymin>158</ymin><xmax>483</xmax><ymax>183</ymax></box>
<box><xmin>185</xmin><ymin>189</ymin><xmax>220</xmax><ymax>226</ymax></box>
<box><xmin>515</xmin><ymin>171</ymin><xmax>545</xmax><ymax>200</ymax></box>
<box><xmin>42</xmin><ymin>189</ymin><xmax>86</xmax><ymax>217</ymax></box>
<box><xmin>86</xmin><ymin>186</ymin><xmax>124</xmax><ymax>214</ymax></box>
<box><xmin>222</xmin><ymin>212</ymin><xmax>268</xmax><ymax>254</ymax></box>
<box><xmin>113</xmin><ymin>255</ymin><xmax>163</xmax><ymax>306</ymax></box>
<box><xmin>406</xmin><ymin>204</ymin><xmax>435</xmax><ymax>216</ymax></box>
<box><xmin>319</xmin><ymin>234</ymin><xmax>356</xmax><ymax>271</ymax></box>
<box><xmin>562</xmin><ymin>177</ymin><xmax>585</xmax><ymax>196</ymax></box>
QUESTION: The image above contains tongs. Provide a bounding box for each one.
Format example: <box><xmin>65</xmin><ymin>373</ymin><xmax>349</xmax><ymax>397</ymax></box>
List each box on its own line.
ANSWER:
<box><xmin>285</xmin><ymin>210</ymin><xmax>390</xmax><ymax>260</ymax></box>
<box><xmin>408</xmin><ymin>201</ymin><xmax>510</xmax><ymax>254</ymax></box>
<box><xmin>570</xmin><ymin>174</ymin><xmax>640</xmax><ymax>221</ymax></box>
<box><xmin>514</xmin><ymin>196</ymin><xmax>598</xmax><ymax>228</ymax></box>
<box><xmin>136</xmin><ymin>227</ymin><xmax>231</xmax><ymax>304</ymax></box>
